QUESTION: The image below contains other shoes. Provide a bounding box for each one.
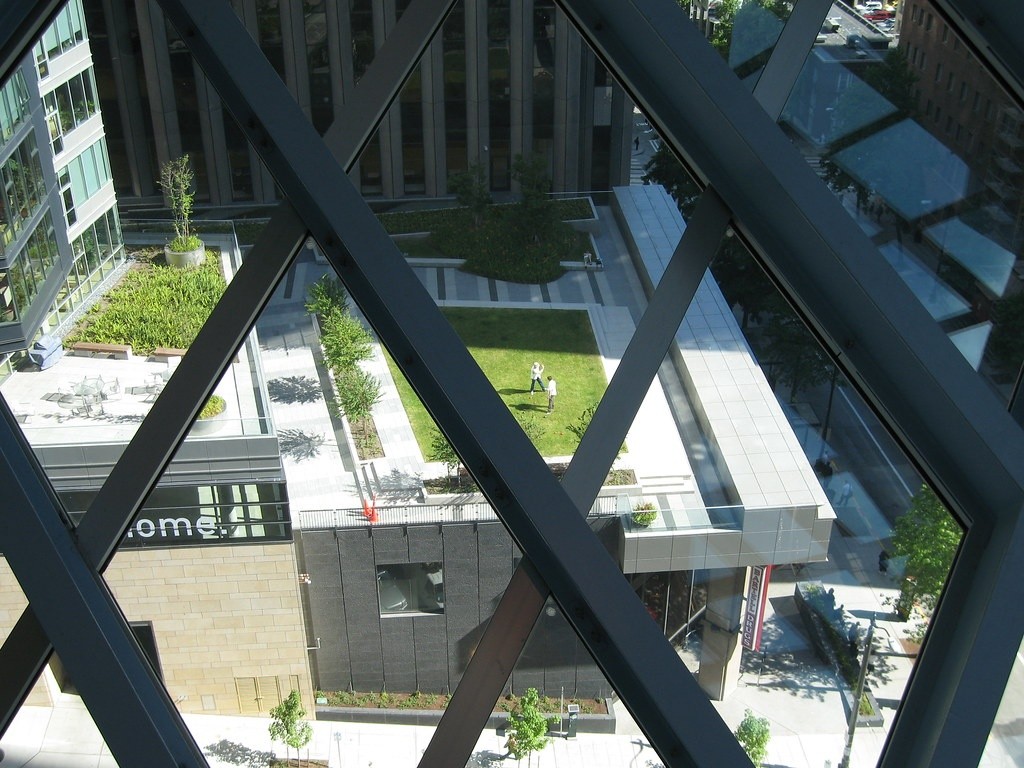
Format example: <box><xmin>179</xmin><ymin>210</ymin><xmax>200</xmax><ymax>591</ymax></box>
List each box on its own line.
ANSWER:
<box><xmin>546</xmin><ymin>412</ymin><xmax>550</xmax><ymax>414</ymax></box>
<box><xmin>545</xmin><ymin>388</ymin><xmax>549</xmax><ymax>391</ymax></box>
<box><xmin>530</xmin><ymin>391</ymin><xmax>533</xmax><ymax>396</ymax></box>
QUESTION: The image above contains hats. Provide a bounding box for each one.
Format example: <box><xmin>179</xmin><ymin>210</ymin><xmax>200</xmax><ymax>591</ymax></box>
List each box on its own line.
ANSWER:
<box><xmin>534</xmin><ymin>362</ymin><xmax>538</xmax><ymax>367</ymax></box>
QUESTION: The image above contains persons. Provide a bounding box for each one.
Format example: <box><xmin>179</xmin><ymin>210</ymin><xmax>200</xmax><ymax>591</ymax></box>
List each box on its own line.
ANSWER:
<box><xmin>634</xmin><ymin>137</ymin><xmax>640</xmax><ymax>150</ymax></box>
<box><xmin>827</xmin><ymin>587</ymin><xmax>876</xmax><ymax>676</ymax></box>
<box><xmin>530</xmin><ymin>362</ymin><xmax>547</xmax><ymax>396</ymax></box>
<box><xmin>496</xmin><ymin>732</ymin><xmax>522</xmax><ymax>762</ymax></box>
<box><xmin>878</xmin><ymin>549</ymin><xmax>891</xmax><ymax>577</ymax></box>
<box><xmin>583</xmin><ymin>249</ymin><xmax>592</xmax><ymax>269</ymax></box>
<box><xmin>546</xmin><ymin>375</ymin><xmax>557</xmax><ymax>415</ymax></box>
<box><xmin>821</xmin><ymin>450</ymin><xmax>854</xmax><ymax>506</ymax></box>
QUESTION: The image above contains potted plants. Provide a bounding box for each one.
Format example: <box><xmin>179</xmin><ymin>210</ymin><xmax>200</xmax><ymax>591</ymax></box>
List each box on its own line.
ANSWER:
<box><xmin>191</xmin><ymin>395</ymin><xmax>228</xmax><ymax>435</ymax></box>
<box><xmin>0</xmin><ymin>92</ymin><xmax>109</xmax><ymax>323</ymax></box>
<box><xmin>631</xmin><ymin>505</ymin><xmax>658</xmax><ymax>530</ymax></box>
<box><xmin>155</xmin><ymin>154</ymin><xmax>204</xmax><ymax>267</ymax></box>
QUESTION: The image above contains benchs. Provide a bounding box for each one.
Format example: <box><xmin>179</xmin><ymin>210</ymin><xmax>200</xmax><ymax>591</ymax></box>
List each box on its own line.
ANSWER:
<box><xmin>154</xmin><ymin>348</ymin><xmax>190</xmax><ymax>363</ymax></box>
<box><xmin>73</xmin><ymin>341</ymin><xmax>132</xmax><ymax>359</ymax></box>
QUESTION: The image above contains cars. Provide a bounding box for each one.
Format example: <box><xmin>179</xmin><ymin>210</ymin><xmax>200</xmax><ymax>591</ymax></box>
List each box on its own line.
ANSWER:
<box><xmin>854</xmin><ymin>0</ymin><xmax>895</xmax><ymax>33</ymax></box>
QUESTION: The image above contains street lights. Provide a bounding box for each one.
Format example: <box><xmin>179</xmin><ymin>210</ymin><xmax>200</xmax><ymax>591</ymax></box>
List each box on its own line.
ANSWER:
<box><xmin>838</xmin><ymin>610</ymin><xmax>897</xmax><ymax>768</ymax></box>
